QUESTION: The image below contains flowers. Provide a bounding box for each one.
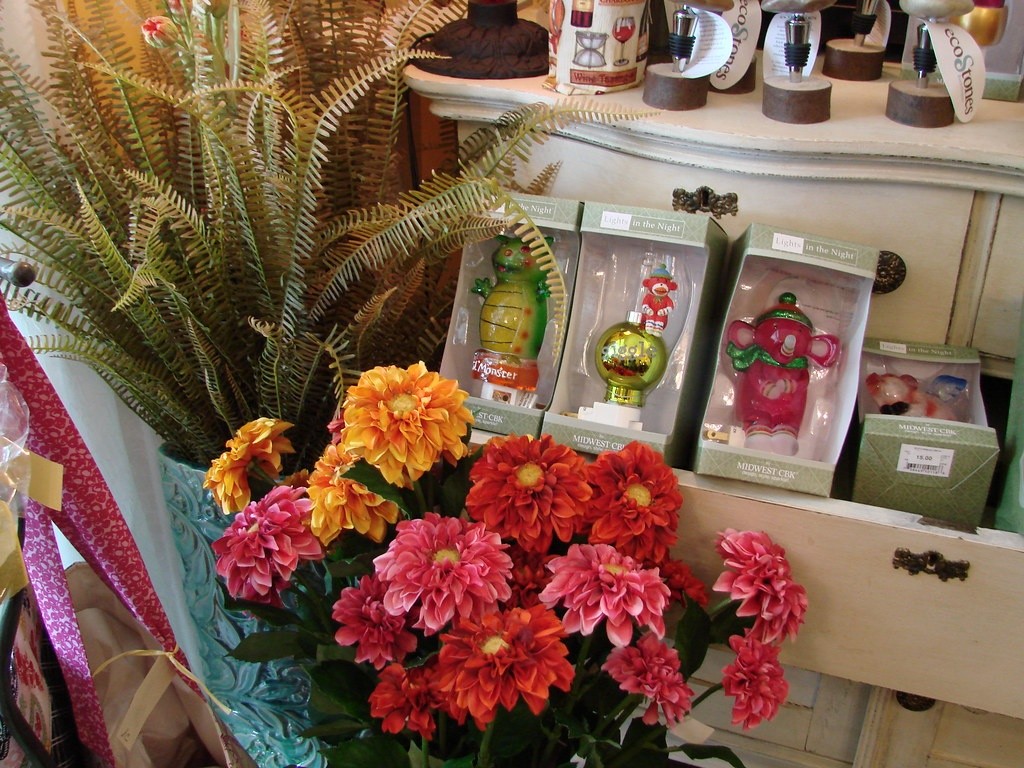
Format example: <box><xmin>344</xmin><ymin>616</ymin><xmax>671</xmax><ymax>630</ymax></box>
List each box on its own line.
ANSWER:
<box><xmin>199</xmin><ymin>359</ymin><xmax>809</xmax><ymax>768</ymax></box>
<box><xmin>0</xmin><ymin>0</ymin><xmax>668</xmax><ymax>481</ymax></box>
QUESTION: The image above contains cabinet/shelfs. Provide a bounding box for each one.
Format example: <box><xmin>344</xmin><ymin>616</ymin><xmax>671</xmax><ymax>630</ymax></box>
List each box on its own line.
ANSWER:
<box><xmin>402</xmin><ymin>65</ymin><xmax>1024</xmax><ymax>768</ymax></box>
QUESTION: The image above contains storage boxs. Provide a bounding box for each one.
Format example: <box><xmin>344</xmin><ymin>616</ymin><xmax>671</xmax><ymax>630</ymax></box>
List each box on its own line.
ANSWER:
<box><xmin>435</xmin><ymin>184</ymin><xmax>584</xmax><ymax>441</ymax></box>
<box><xmin>683</xmin><ymin>221</ymin><xmax>880</xmax><ymax>499</ymax></box>
<box><xmin>841</xmin><ymin>333</ymin><xmax>999</xmax><ymax>527</ymax></box>
<box><xmin>535</xmin><ymin>197</ymin><xmax>730</xmax><ymax>467</ymax></box>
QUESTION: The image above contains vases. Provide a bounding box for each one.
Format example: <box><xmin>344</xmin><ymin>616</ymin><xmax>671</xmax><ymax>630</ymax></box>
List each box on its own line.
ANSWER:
<box><xmin>158</xmin><ymin>440</ymin><xmax>380</xmax><ymax>768</ymax></box>
